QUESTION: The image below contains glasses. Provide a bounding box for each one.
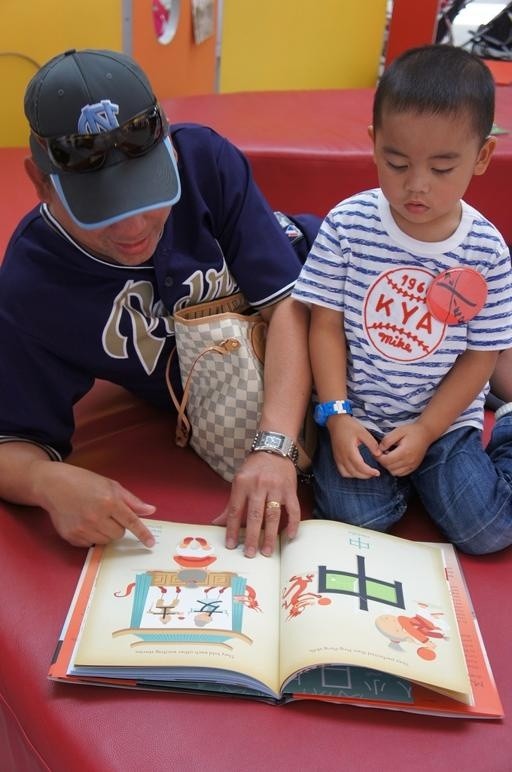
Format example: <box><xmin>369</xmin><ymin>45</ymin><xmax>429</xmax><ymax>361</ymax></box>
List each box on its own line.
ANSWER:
<box><xmin>36</xmin><ymin>105</ymin><xmax>167</xmax><ymax>169</ymax></box>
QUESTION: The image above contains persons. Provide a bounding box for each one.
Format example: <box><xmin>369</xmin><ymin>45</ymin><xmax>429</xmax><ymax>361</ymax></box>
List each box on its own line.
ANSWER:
<box><xmin>294</xmin><ymin>41</ymin><xmax>512</xmax><ymax>555</ymax></box>
<box><xmin>0</xmin><ymin>49</ymin><xmax>326</xmax><ymax>554</ymax></box>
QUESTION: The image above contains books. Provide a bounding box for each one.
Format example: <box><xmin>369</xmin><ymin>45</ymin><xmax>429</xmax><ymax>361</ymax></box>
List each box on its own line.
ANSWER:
<box><xmin>44</xmin><ymin>516</ymin><xmax>506</xmax><ymax>723</ymax></box>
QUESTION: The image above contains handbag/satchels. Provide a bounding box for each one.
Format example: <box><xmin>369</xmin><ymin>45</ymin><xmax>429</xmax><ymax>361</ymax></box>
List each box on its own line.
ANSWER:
<box><xmin>166</xmin><ymin>292</ymin><xmax>321</xmax><ymax>482</ymax></box>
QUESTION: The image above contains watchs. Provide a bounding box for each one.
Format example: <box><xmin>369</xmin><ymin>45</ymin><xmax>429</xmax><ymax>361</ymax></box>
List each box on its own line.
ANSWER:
<box><xmin>252</xmin><ymin>431</ymin><xmax>300</xmax><ymax>463</ymax></box>
<box><xmin>314</xmin><ymin>398</ymin><xmax>352</xmax><ymax>423</ymax></box>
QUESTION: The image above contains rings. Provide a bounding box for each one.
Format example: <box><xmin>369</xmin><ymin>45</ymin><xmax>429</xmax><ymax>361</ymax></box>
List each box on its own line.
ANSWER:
<box><xmin>266</xmin><ymin>501</ymin><xmax>282</xmax><ymax>514</ymax></box>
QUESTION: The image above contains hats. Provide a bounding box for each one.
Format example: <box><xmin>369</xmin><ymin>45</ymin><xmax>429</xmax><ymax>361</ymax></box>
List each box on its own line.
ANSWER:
<box><xmin>23</xmin><ymin>51</ymin><xmax>183</xmax><ymax>229</ymax></box>
